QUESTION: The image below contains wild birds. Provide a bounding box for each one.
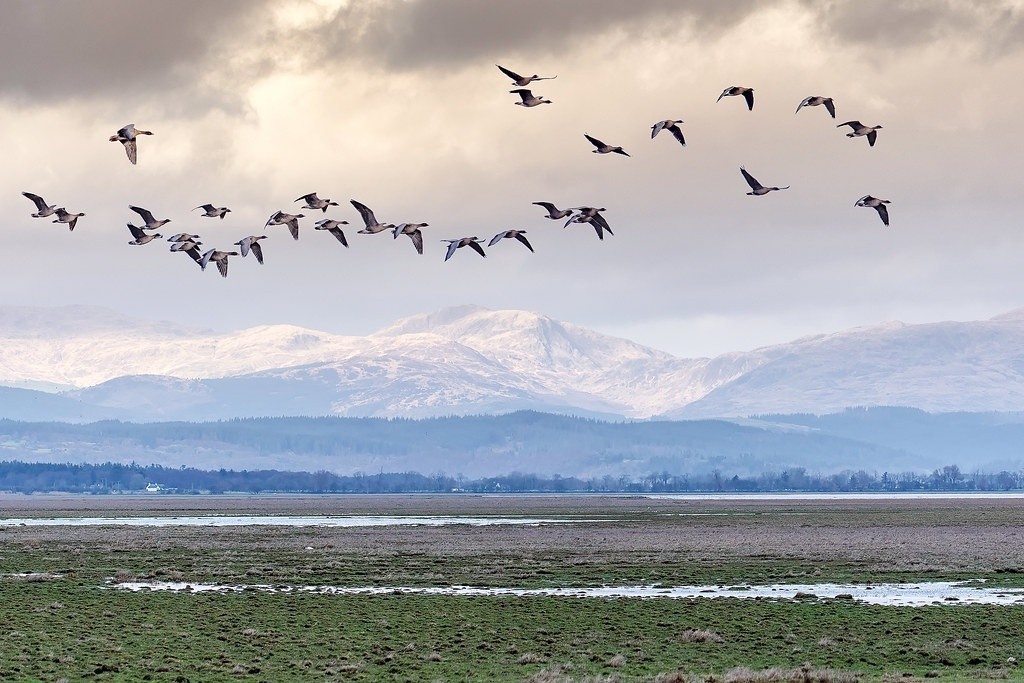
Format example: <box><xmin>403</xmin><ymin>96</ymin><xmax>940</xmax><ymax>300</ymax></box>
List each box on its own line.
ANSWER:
<box><xmin>234</xmin><ymin>235</ymin><xmax>267</xmax><ymax>264</ymax></box>
<box><xmin>488</xmin><ymin>230</ymin><xmax>535</xmax><ymax>253</ymax></box>
<box><xmin>109</xmin><ymin>123</ymin><xmax>154</xmax><ymax>165</ymax></box>
<box><xmin>295</xmin><ymin>192</ymin><xmax>339</xmax><ymax>213</ymax></box>
<box><xmin>585</xmin><ymin>134</ymin><xmax>630</xmax><ymax>156</ymax></box>
<box><xmin>21</xmin><ymin>192</ymin><xmax>86</xmax><ymax>231</ymax></box>
<box><xmin>651</xmin><ymin>119</ymin><xmax>686</xmax><ymax>146</ymax></box>
<box><xmin>192</xmin><ymin>204</ymin><xmax>232</xmax><ymax>219</ymax></box>
<box><xmin>315</xmin><ymin>218</ymin><xmax>349</xmax><ymax>247</ymax></box>
<box><xmin>126</xmin><ymin>205</ymin><xmax>171</xmax><ymax>245</ymax></box>
<box><xmin>532</xmin><ymin>201</ymin><xmax>614</xmax><ymax>241</ymax></box>
<box><xmin>509</xmin><ymin>89</ymin><xmax>553</xmax><ymax>107</ymax></box>
<box><xmin>263</xmin><ymin>209</ymin><xmax>305</xmax><ymax>240</ymax></box>
<box><xmin>740</xmin><ymin>168</ymin><xmax>791</xmax><ymax>196</ymax></box>
<box><xmin>795</xmin><ymin>96</ymin><xmax>836</xmax><ymax>118</ymax></box>
<box><xmin>441</xmin><ymin>236</ymin><xmax>487</xmax><ymax>261</ymax></box>
<box><xmin>717</xmin><ymin>86</ymin><xmax>754</xmax><ymax>110</ymax></box>
<box><xmin>854</xmin><ymin>195</ymin><xmax>891</xmax><ymax>226</ymax></box>
<box><xmin>837</xmin><ymin>121</ymin><xmax>882</xmax><ymax>147</ymax></box>
<box><xmin>166</xmin><ymin>232</ymin><xmax>203</xmax><ymax>267</ymax></box>
<box><xmin>197</xmin><ymin>248</ymin><xmax>239</xmax><ymax>278</ymax></box>
<box><xmin>495</xmin><ymin>64</ymin><xmax>557</xmax><ymax>87</ymax></box>
<box><xmin>350</xmin><ymin>199</ymin><xmax>396</xmax><ymax>234</ymax></box>
<box><xmin>392</xmin><ymin>222</ymin><xmax>430</xmax><ymax>255</ymax></box>
